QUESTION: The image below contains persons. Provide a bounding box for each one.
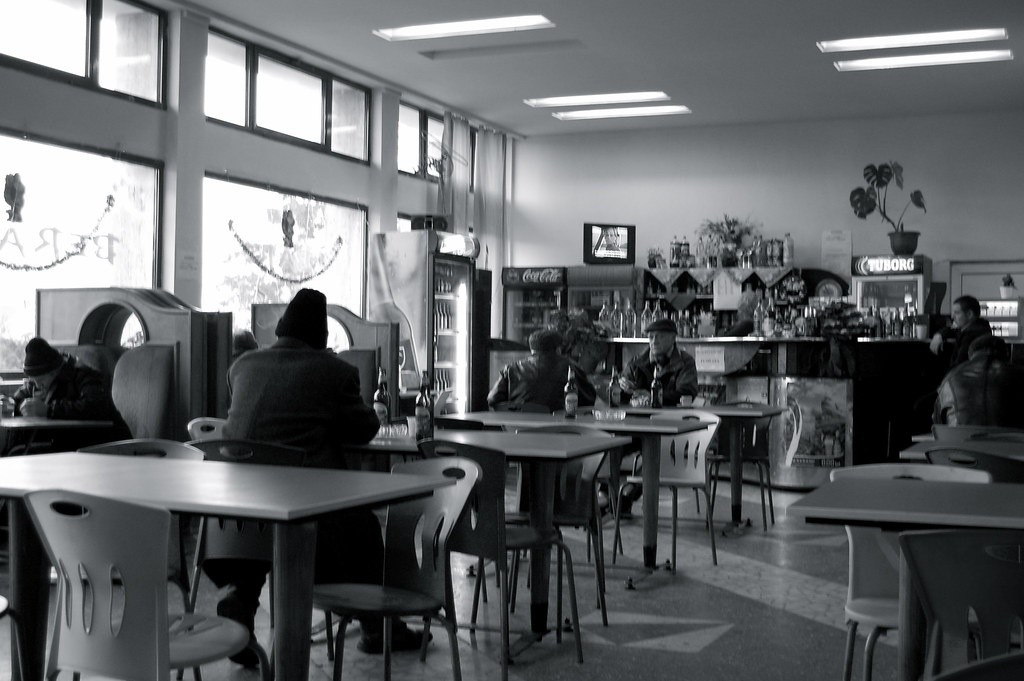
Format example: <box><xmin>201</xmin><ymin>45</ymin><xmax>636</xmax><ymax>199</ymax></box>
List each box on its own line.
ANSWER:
<box><xmin>593</xmin><ymin>320</ymin><xmax>697</xmax><ymax>520</ymax></box>
<box><xmin>486</xmin><ymin>329</ymin><xmax>610</xmax><ymax>529</ymax></box>
<box><xmin>933</xmin><ymin>336</ymin><xmax>1024</xmax><ymax>463</ymax></box>
<box><xmin>11</xmin><ymin>338</ymin><xmax>135</xmax><ymax>514</ymax></box>
<box><xmin>603</xmin><ymin>227</ymin><xmax>621</xmax><ymax>258</ymax></box>
<box><xmin>231</xmin><ymin>332</ymin><xmax>261</xmax><ymax>361</ymax></box>
<box><xmin>930</xmin><ymin>296</ymin><xmax>991</xmax><ymax>367</ymax></box>
<box><xmin>215</xmin><ymin>288</ymin><xmax>433</xmax><ymax>669</ymax></box>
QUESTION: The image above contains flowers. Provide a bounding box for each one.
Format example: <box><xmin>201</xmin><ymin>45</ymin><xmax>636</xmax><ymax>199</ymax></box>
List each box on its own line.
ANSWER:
<box><xmin>694</xmin><ymin>217</ymin><xmax>753</xmax><ymax>258</ymax></box>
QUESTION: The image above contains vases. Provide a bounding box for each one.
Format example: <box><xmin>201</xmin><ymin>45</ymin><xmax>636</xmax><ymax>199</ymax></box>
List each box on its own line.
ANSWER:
<box><xmin>720</xmin><ymin>244</ymin><xmax>738</xmax><ymax>266</ymax></box>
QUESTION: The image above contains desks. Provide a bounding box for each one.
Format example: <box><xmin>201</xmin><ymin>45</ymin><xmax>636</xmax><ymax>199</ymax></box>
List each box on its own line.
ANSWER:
<box><xmin>789</xmin><ymin>483</ymin><xmax>1024</xmax><ymax>680</ymax></box>
<box><xmin>899</xmin><ymin>439</ymin><xmax>1024</xmax><ymax>466</ymax></box>
<box><xmin>912</xmin><ymin>435</ymin><xmax>936</xmax><ymax>443</ymax></box>
<box><xmin>0</xmin><ymin>416</ymin><xmax>115</xmax><ymax>446</ymax></box>
<box><xmin>1</xmin><ymin>453</ymin><xmax>458</xmax><ymax>681</ymax></box>
<box><xmin>588</xmin><ymin>402</ymin><xmax>787</xmax><ymax>540</ymax></box>
<box><xmin>435</xmin><ymin>410</ymin><xmax>717</xmax><ymax>592</ymax></box>
<box><xmin>367</xmin><ymin>430</ymin><xmax>633</xmax><ymax>681</ymax></box>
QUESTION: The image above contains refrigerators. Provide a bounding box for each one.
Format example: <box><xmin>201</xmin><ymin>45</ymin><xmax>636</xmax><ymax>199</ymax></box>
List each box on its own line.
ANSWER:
<box><xmin>850</xmin><ymin>255</ymin><xmax>933</xmax><ymax>316</ymax></box>
<box><xmin>368</xmin><ymin>232</ymin><xmax>480</xmax><ymax>417</ymax></box>
<box><xmin>566</xmin><ymin>263</ymin><xmax>644</xmax><ymax>339</ymax></box>
<box><xmin>501</xmin><ymin>267</ymin><xmax>566</xmax><ymax>348</ymax></box>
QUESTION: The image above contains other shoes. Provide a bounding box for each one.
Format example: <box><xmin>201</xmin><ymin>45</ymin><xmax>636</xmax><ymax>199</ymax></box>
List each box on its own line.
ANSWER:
<box><xmin>217</xmin><ymin>589</ymin><xmax>269</xmax><ymax>673</ymax></box>
<box><xmin>357</xmin><ymin>619</ymin><xmax>433</xmax><ymax>657</ymax></box>
<box><xmin>618</xmin><ymin>494</ymin><xmax>634</xmax><ymax>521</ymax></box>
<box><xmin>594</xmin><ymin>482</ymin><xmax>610</xmax><ymax>512</ymax></box>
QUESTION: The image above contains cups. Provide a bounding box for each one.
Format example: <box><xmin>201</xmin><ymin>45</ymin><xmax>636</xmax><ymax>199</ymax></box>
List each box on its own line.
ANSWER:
<box><xmin>681</xmin><ymin>396</ymin><xmax>693</xmax><ymax>406</ymax></box>
<box><xmin>407</xmin><ymin>417</ymin><xmax>416</xmax><ymax>437</ymax></box>
<box><xmin>917</xmin><ymin>314</ymin><xmax>930</xmax><ymax>340</ymax></box>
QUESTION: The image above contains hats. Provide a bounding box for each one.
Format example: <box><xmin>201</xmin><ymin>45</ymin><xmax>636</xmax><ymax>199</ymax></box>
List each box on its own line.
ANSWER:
<box><xmin>274</xmin><ymin>288</ymin><xmax>329</xmax><ymax>348</ymax></box>
<box><xmin>528</xmin><ymin>329</ymin><xmax>564</xmax><ymax>352</ymax></box>
<box><xmin>23</xmin><ymin>338</ymin><xmax>62</xmax><ymax>377</ymax></box>
<box><xmin>645</xmin><ymin>320</ymin><xmax>677</xmax><ymax>335</ymax></box>
<box><xmin>967</xmin><ymin>336</ymin><xmax>1007</xmax><ymax>358</ymax></box>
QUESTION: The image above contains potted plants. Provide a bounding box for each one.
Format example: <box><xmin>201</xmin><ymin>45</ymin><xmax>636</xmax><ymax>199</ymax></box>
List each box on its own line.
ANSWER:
<box><xmin>1000</xmin><ymin>274</ymin><xmax>1016</xmax><ymax>300</ymax></box>
<box><xmin>849</xmin><ymin>159</ymin><xmax>928</xmax><ymax>257</ymax></box>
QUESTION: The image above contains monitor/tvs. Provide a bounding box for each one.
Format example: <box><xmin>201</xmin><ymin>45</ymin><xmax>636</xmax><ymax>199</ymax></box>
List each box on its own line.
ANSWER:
<box><xmin>583</xmin><ymin>223</ymin><xmax>636</xmax><ymax>265</ymax></box>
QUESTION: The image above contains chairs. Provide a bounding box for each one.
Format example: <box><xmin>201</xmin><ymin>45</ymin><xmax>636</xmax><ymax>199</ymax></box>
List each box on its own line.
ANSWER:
<box><xmin>1</xmin><ymin>289</ymin><xmax>1024</xmax><ymax>681</ymax></box>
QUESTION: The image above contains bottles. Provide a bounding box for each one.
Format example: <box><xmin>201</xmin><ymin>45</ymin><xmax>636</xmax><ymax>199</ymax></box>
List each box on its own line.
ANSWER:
<box><xmin>369</xmin><ymin>234</ymin><xmax>420</xmax><ymax>390</ymax></box>
<box><xmin>650</xmin><ymin>366</ymin><xmax>663</xmax><ymax>408</ymax></box>
<box><xmin>599</xmin><ymin>280</ymin><xmax>667</xmax><ymax>338</ymax></box>
<box><xmin>415</xmin><ymin>370</ymin><xmax>434</xmax><ymax>447</ymax></box>
<box><xmin>867</xmin><ymin>305</ymin><xmax>914</xmax><ymax>338</ymax></box>
<box><xmin>434</xmin><ymin>369</ymin><xmax>451</xmax><ymax>392</ymax></box>
<box><xmin>670</xmin><ymin>235</ymin><xmax>817</xmax><ymax>337</ymax></box>
<box><xmin>609</xmin><ymin>365</ymin><xmax>620</xmax><ymax>408</ymax></box>
<box><xmin>373</xmin><ymin>367</ymin><xmax>392</xmax><ymax>424</ymax></box>
<box><xmin>435</xmin><ymin>264</ymin><xmax>455</xmax><ymax>293</ymax></box>
<box><xmin>435</xmin><ymin>302</ymin><xmax>452</xmax><ymax>330</ymax></box>
<box><xmin>564</xmin><ymin>365</ymin><xmax>577</xmax><ymax>419</ymax></box>
